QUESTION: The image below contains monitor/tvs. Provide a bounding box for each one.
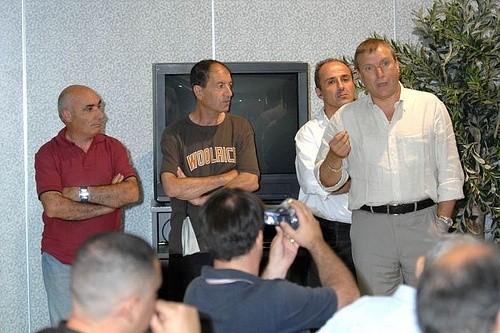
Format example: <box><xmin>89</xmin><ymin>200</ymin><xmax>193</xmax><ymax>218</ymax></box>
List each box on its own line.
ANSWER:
<box><xmin>152</xmin><ymin>62</ymin><xmax>308</xmax><ymax>202</ymax></box>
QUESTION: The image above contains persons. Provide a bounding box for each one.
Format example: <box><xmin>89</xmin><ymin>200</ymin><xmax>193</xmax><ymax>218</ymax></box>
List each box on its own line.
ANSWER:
<box><xmin>184</xmin><ymin>186</ymin><xmax>360</xmax><ymax>333</ymax></box>
<box><xmin>33</xmin><ymin>231</ymin><xmax>200</xmax><ymax>333</ymax></box>
<box><xmin>267</xmin><ymin>212</ymin><xmax>275</xmax><ymax>224</ymax></box>
<box><xmin>313</xmin><ymin>38</ymin><xmax>466</xmax><ymax>297</ymax></box>
<box><xmin>416</xmin><ymin>240</ymin><xmax>500</xmax><ymax>333</ymax></box>
<box><xmin>33</xmin><ymin>84</ymin><xmax>139</xmax><ymax>327</ymax></box>
<box><xmin>317</xmin><ymin>233</ymin><xmax>477</xmax><ymax>333</ymax></box>
<box><xmin>294</xmin><ymin>59</ymin><xmax>356</xmax><ymax>288</ymax></box>
<box><xmin>253</xmin><ymin>80</ymin><xmax>297</xmax><ymax>174</ymax></box>
<box><xmin>161</xmin><ymin>59</ymin><xmax>260</xmax><ymax>303</ymax></box>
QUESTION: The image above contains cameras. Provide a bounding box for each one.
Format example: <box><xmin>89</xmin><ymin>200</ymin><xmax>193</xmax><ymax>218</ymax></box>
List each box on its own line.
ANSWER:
<box><xmin>263</xmin><ymin>198</ymin><xmax>297</xmax><ymax>225</ymax></box>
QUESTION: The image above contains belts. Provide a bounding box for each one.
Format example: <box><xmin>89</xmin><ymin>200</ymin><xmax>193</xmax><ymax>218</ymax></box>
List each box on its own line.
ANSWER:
<box><xmin>360</xmin><ymin>198</ymin><xmax>435</xmax><ymax>215</ymax></box>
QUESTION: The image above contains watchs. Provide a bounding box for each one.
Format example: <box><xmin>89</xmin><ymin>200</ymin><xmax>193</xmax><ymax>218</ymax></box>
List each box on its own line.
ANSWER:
<box><xmin>439</xmin><ymin>214</ymin><xmax>453</xmax><ymax>226</ymax></box>
<box><xmin>79</xmin><ymin>186</ymin><xmax>88</xmax><ymax>203</ymax></box>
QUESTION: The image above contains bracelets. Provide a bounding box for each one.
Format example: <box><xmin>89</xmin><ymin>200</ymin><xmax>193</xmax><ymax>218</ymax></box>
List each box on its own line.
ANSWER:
<box><xmin>325</xmin><ymin>161</ymin><xmax>342</xmax><ymax>172</ymax></box>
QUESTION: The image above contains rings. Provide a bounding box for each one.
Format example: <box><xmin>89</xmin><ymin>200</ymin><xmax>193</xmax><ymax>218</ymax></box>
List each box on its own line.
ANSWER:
<box><xmin>290</xmin><ymin>239</ymin><xmax>295</xmax><ymax>242</ymax></box>
